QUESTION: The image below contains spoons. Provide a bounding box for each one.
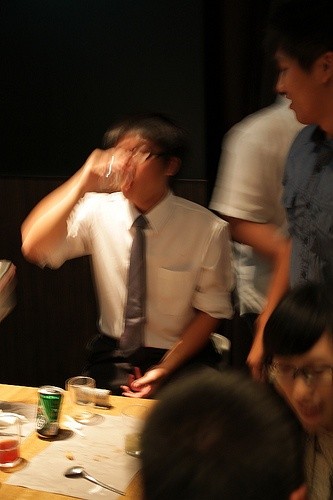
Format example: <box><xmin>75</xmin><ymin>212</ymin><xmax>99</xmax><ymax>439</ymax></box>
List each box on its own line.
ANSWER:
<box><xmin>65</xmin><ymin>466</ymin><xmax>127</xmax><ymax>496</ymax></box>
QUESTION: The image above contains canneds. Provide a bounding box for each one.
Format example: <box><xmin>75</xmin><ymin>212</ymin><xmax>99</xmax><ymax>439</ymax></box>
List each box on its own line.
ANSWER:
<box><xmin>34</xmin><ymin>386</ymin><xmax>64</xmax><ymax>438</ymax></box>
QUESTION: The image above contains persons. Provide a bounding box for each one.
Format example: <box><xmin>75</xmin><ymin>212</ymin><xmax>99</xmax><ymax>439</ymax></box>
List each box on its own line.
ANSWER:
<box><xmin>209</xmin><ymin>27</ymin><xmax>333</xmax><ymax>379</ymax></box>
<box><xmin>22</xmin><ymin>120</ymin><xmax>236</xmax><ymax>399</ymax></box>
<box><xmin>138</xmin><ymin>281</ymin><xmax>333</xmax><ymax>500</ymax></box>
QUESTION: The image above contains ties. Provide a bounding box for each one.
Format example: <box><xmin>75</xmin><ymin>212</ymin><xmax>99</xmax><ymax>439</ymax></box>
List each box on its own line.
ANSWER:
<box><xmin>120</xmin><ymin>215</ymin><xmax>147</xmax><ymax>359</ymax></box>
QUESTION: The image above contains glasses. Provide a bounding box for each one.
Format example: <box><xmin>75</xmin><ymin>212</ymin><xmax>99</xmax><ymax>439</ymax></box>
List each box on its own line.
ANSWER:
<box><xmin>267</xmin><ymin>362</ymin><xmax>333</xmax><ymax>387</ymax></box>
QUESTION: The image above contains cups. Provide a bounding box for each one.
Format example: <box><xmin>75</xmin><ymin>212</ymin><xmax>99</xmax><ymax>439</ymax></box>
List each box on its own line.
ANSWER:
<box><xmin>122</xmin><ymin>405</ymin><xmax>150</xmax><ymax>457</ymax></box>
<box><xmin>0</xmin><ymin>413</ymin><xmax>23</xmax><ymax>473</ymax></box>
<box><xmin>68</xmin><ymin>376</ymin><xmax>96</xmax><ymax>424</ymax></box>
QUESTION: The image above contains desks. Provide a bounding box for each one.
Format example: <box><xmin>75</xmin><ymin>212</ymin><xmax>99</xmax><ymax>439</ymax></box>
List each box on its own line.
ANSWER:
<box><xmin>1</xmin><ymin>383</ymin><xmax>159</xmax><ymax>500</ymax></box>
